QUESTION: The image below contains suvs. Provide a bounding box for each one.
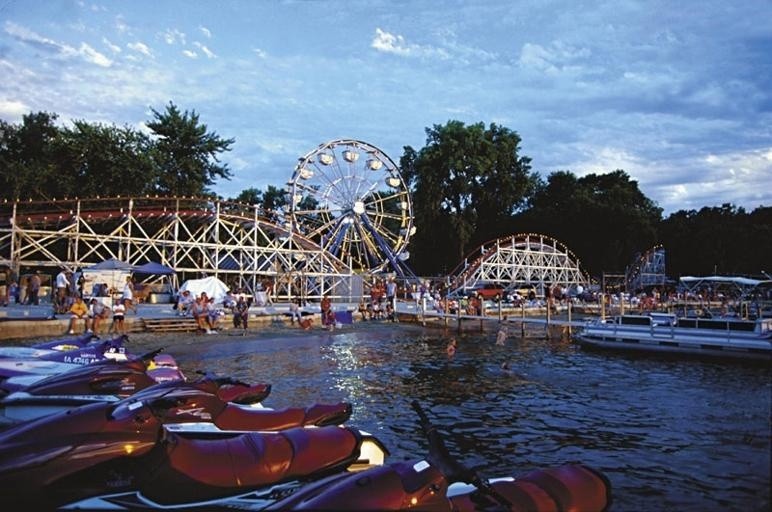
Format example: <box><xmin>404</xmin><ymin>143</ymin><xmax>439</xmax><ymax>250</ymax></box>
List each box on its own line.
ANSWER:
<box><xmin>502</xmin><ymin>284</ymin><xmax>537</xmax><ymax>303</ymax></box>
<box><xmin>579</xmin><ymin>284</ymin><xmax>603</xmax><ymax>302</ymax></box>
<box><xmin>461</xmin><ymin>282</ymin><xmax>508</xmax><ymax>301</ymax></box>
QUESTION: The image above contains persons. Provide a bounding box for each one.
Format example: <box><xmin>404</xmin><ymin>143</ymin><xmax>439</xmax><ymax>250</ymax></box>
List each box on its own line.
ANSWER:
<box><xmin>507</xmin><ymin>284</ymin><xmax>597</xmax><ymax>312</ymax></box>
<box><xmin>613</xmin><ymin>288</ymin><xmax>660</xmax><ymax>312</ymax></box>
<box><xmin>256</xmin><ymin>276</ymin><xmax>274</xmax><ymax>307</ymax></box>
<box><xmin>1</xmin><ymin>266</ymin><xmax>41</xmax><ymax>308</ymax></box>
<box><xmin>290</xmin><ymin>294</ymin><xmax>336</xmax><ymax>331</ymax></box>
<box><xmin>418</xmin><ymin>280</ymin><xmax>481</xmax><ymax>317</ymax></box>
<box><xmin>52</xmin><ymin>268</ymin><xmax>137</xmax><ymax>337</ymax></box>
<box><xmin>359</xmin><ymin>276</ymin><xmax>396</xmax><ymax>320</ymax></box>
<box><xmin>176</xmin><ymin>289</ymin><xmax>250</xmax><ymax>334</ymax></box>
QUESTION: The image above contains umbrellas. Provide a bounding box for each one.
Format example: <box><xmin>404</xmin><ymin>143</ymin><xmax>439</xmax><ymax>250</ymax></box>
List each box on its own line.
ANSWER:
<box><xmin>88</xmin><ymin>257</ymin><xmax>136</xmax><ymax>292</ymax></box>
<box><xmin>136</xmin><ymin>260</ymin><xmax>175</xmax><ymax>286</ymax></box>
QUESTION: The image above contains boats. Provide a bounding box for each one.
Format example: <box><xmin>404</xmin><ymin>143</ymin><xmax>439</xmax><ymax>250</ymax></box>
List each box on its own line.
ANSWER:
<box><xmin>571</xmin><ymin>275</ymin><xmax>771</xmax><ymax>362</ymax></box>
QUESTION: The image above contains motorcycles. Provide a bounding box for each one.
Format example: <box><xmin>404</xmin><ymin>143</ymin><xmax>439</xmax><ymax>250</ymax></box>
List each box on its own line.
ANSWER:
<box><xmin>0</xmin><ymin>334</ymin><xmax>614</xmax><ymax>512</ymax></box>
